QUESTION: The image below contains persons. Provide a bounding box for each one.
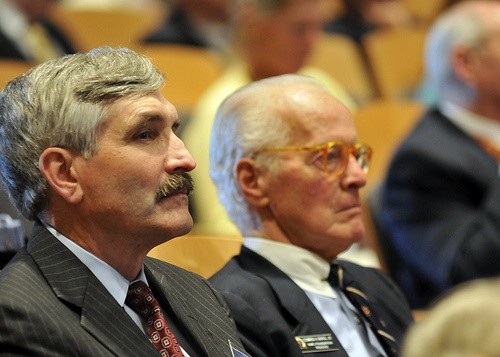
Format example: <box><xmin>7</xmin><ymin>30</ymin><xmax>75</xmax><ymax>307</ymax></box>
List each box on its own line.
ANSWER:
<box><xmin>0</xmin><ymin>45</ymin><xmax>253</xmax><ymax>357</ymax></box>
<box><xmin>204</xmin><ymin>72</ymin><xmax>419</xmax><ymax>357</ymax></box>
<box><xmin>182</xmin><ymin>0</ymin><xmax>382</xmax><ymax>266</ymax></box>
<box><xmin>0</xmin><ymin>0</ymin><xmax>404</xmax><ymax>96</ymax></box>
<box><xmin>370</xmin><ymin>0</ymin><xmax>499</xmax><ymax>311</ymax></box>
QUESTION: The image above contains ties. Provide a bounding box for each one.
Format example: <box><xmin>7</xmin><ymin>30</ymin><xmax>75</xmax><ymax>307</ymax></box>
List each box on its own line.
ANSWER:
<box><xmin>327</xmin><ymin>263</ymin><xmax>401</xmax><ymax>357</ymax></box>
<box><xmin>123</xmin><ymin>279</ymin><xmax>185</xmax><ymax>357</ymax></box>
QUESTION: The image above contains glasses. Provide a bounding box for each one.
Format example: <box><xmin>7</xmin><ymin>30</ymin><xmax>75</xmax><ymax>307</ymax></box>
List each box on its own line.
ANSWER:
<box><xmin>249</xmin><ymin>137</ymin><xmax>373</xmax><ymax>178</ymax></box>
